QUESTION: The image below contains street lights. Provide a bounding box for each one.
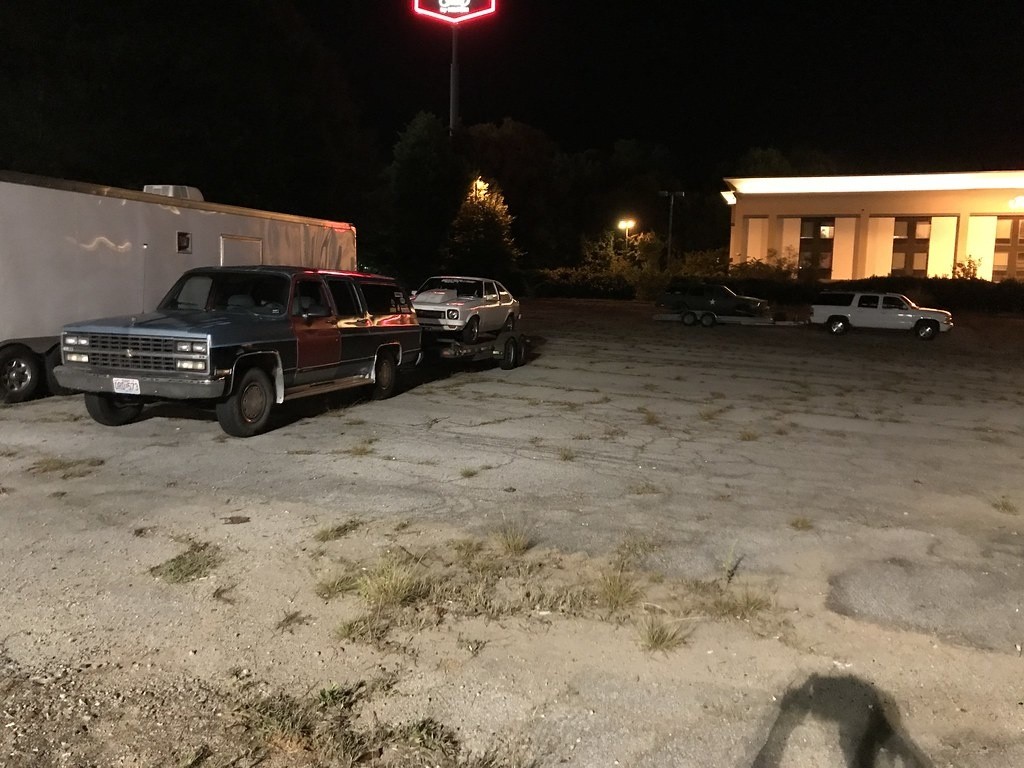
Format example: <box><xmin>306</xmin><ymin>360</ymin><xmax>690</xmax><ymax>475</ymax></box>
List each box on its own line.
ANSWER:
<box><xmin>657</xmin><ymin>190</ymin><xmax>685</xmax><ymax>274</ymax></box>
<box><xmin>619</xmin><ymin>221</ymin><xmax>635</xmax><ymax>253</ymax></box>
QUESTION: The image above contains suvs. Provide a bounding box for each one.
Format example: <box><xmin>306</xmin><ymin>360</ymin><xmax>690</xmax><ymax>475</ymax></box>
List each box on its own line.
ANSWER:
<box><xmin>50</xmin><ymin>262</ymin><xmax>424</xmax><ymax>438</ymax></box>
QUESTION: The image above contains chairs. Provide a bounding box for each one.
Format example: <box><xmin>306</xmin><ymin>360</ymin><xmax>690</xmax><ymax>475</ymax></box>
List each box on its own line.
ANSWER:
<box><xmin>227</xmin><ymin>295</ymin><xmax>254</xmax><ymax>308</ymax></box>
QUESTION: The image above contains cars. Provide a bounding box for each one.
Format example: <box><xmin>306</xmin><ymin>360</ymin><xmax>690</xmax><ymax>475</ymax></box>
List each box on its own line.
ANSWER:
<box><xmin>654</xmin><ymin>280</ymin><xmax>771</xmax><ymax>316</ymax></box>
<box><xmin>407</xmin><ymin>275</ymin><xmax>522</xmax><ymax>343</ymax></box>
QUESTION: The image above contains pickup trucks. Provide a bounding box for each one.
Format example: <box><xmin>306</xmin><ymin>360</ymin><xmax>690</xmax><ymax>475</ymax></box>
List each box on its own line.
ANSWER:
<box><xmin>808</xmin><ymin>290</ymin><xmax>956</xmax><ymax>340</ymax></box>
